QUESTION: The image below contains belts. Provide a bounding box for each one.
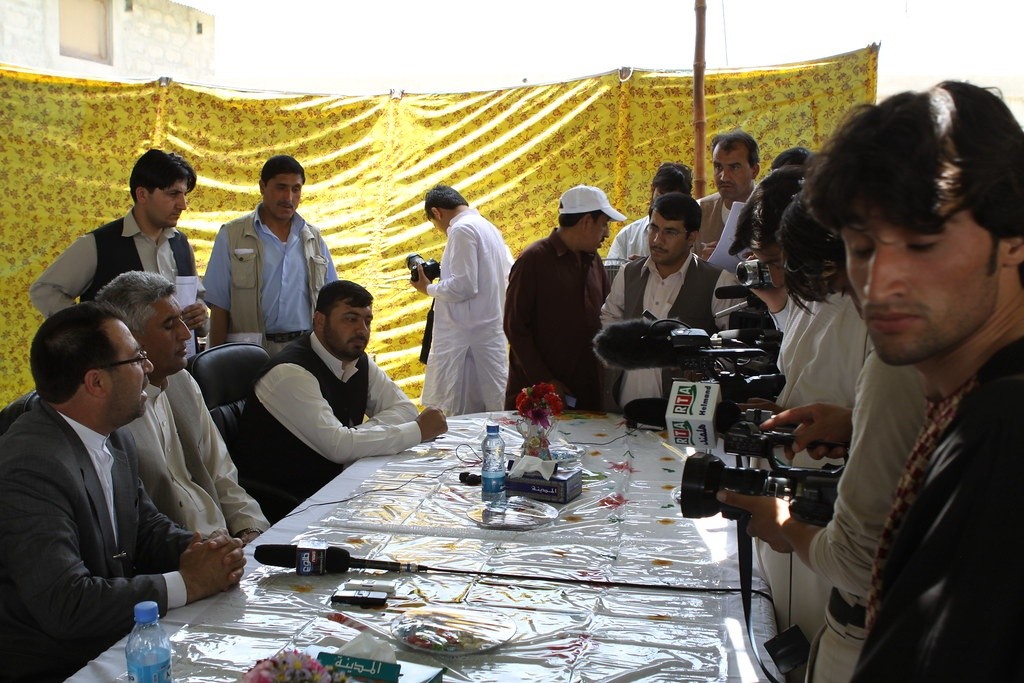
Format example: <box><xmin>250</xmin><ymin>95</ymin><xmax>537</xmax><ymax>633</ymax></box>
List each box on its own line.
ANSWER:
<box><xmin>827</xmin><ymin>587</ymin><xmax>867</xmax><ymax>629</ymax></box>
<box><xmin>265</xmin><ymin>330</ymin><xmax>311</xmax><ymax>343</ymax></box>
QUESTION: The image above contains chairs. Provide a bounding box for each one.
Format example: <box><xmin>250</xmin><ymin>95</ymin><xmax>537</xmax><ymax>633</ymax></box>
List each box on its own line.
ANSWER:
<box><xmin>188</xmin><ymin>341</ymin><xmax>271</xmax><ymax>454</ymax></box>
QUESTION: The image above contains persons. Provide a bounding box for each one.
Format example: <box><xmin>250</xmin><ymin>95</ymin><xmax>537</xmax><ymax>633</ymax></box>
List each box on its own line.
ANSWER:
<box><xmin>696</xmin><ymin>132</ymin><xmax>759</xmax><ymax>259</ymax></box>
<box><xmin>93</xmin><ymin>269</ymin><xmax>271</xmax><ymax>545</ymax></box>
<box><xmin>29</xmin><ymin>149</ymin><xmax>209</xmax><ymax>358</ymax></box>
<box><xmin>410</xmin><ymin>184</ymin><xmax>514</xmax><ymax>416</ymax></box>
<box><xmin>598</xmin><ymin>193</ymin><xmax>744</xmax><ymax>425</ymax></box>
<box><xmin>1</xmin><ymin>303</ymin><xmax>247</xmax><ymax>683</ymax></box>
<box><xmin>240</xmin><ymin>280</ymin><xmax>449</xmax><ymax>524</ymax></box>
<box><xmin>716</xmin><ymin>148</ymin><xmax>931</xmax><ymax>683</ymax></box>
<box><xmin>805</xmin><ymin>80</ymin><xmax>1023</xmax><ymax>682</ymax></box>
<box><xmin>605</xmin><ymin>162</ymin><xmax>694</xmax><ymax>267</ymax></box>
<box><xmin>202</xmin><ymin>155</ymin><xmax>338</xmax><ymax>352</ymax></box>
<box><xmin>502</xmin><ymin>187</ymin><xmax>628</xmax><ymax>410</ymax></box>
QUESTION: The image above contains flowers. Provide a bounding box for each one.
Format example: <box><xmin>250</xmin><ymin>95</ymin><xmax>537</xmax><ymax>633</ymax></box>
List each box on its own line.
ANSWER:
<box><xmin>515</xmin><ymin>383</ymin><xmax>562</xmax><ymax>421</ymax></box>
<box><xmin>239</xmin><ymin>648</ymin><xmax>355</xmax><ymax>683</ymax></box>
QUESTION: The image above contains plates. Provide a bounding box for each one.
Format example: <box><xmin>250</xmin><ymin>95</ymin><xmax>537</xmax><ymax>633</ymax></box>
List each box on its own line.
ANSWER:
<box><xmin>466</xmin><ymin>498</ymin><xmax>559</xmax><ymax>530</ymax></box>
<box><xmin>390</xmin><ymin>606</ymin><xmax>517</xmax><ymax>657</ymax></box>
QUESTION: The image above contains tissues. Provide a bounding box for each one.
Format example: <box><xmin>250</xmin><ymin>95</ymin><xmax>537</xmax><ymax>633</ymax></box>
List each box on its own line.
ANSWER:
<box><xmin>505</xmin><ymin>455</ymin><xmax>583</xmax><ymax>505</ymax></box>
<box><xmin>316</xmin><ymin>632</ymin><xmax>444</xmax><ymax>683</ymax></box>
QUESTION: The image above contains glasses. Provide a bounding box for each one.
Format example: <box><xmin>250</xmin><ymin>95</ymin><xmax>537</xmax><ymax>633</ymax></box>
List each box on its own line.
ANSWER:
<box><xmin>658</xmin><ymin>162</ymin><xmax>692</xmax><ymax>176</ymax></box>
<box><xmin>82</xmin><ymin>349</ymin><xmax>148</xmax><ymax>385</ymax></box>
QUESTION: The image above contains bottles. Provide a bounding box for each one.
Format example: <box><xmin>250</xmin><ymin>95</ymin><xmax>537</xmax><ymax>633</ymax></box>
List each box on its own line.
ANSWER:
<box><xmin>124</xmin><ymin>600</ymin><xmax>176</xmax><ymax>683</ymax></box>
<box><xmin>480</xmin><ymin>424</ymin><xmax>506</xmax><ymax>502</ymax></box>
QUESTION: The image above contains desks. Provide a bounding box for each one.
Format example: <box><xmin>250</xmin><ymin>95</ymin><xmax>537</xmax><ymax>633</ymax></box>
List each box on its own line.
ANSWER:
<box><xmin>62</xmin><ymin>411</ymin><xmax>788</xmax><ymax>683</ymax></box>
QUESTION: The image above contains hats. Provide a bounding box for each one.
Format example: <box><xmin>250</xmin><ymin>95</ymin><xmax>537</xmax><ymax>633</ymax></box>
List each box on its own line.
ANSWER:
<box><xmin>558</xmin><ymin>184</ymin><xmax>628</xmax><ymax>222</ymax></box>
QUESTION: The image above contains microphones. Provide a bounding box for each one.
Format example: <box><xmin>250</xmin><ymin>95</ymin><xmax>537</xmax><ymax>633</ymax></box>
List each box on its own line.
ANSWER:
<box><xmin>593</xmin><ymin>317</ymin><xmax>699</xmax><ymax>371</ymax></box>
<box><xmin>254</xmin><ymin>545</ymin><xmax>428</xmax><ymax>578</ymax></box>
<box><xmin>715</xmin><ymin>285</ymin><xmax>752</xmax><ymax>299</ymax></box>
<box><xmin>623</xmin><ymin>381</ymin><xmax>796</xmax><ymax>449</ymax></box>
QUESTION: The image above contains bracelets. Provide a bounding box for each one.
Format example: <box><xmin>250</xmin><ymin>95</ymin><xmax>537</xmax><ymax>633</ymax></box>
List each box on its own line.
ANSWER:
<box><xmin>245</xmin><ymin>528</ymin><xmax>261</xmax><ymax>534</ymax></box>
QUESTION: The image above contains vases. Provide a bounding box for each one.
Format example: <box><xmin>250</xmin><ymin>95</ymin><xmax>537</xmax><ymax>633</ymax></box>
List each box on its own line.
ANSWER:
<box><xmin>517</xmin><ymin>415</ymin><xmax>557</xmax><ymax>462</ymax></box>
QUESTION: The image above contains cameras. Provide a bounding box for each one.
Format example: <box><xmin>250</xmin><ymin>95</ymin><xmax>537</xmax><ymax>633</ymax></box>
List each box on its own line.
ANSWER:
<box><xmin>406</xmin><ymin>254</ymin><xmax>441</xmax><ymax>282</ymax></box>
<box><xmin>736</xmin><ymin>259</ymin><xmax>774</xmax><ymax>288</ymax></box>
